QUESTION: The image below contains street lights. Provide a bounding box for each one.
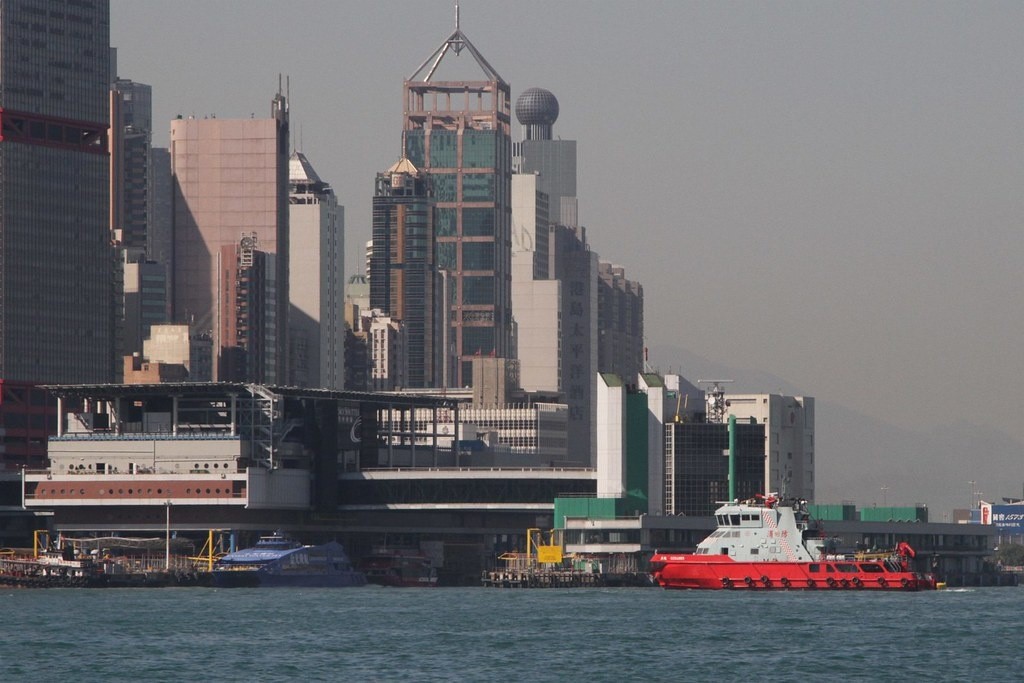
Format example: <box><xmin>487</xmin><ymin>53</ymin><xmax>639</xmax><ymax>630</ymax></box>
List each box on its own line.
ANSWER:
<box><xmin>968</xmin><ymin>480</ymin><xmax>984</xmax><ymax>509</ymax></box>
<box><xmin>881</xmin><ymin>485</ymin><xmax>889</xmax><ymax>506</ymax></box>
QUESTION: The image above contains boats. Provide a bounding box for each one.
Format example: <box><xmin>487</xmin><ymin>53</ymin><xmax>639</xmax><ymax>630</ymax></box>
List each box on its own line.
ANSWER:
<box><xmin>358</xmin><ymin>531</ymin><xmax>438</xmax><ymax>586</ymax></box>
<box><xmin>196</xmin><ymin>532</ymin><xmax>369</xmax><ymax>588</ymax></box>
<box><xmin>650</xmin><ymin>492</ymin><xmax>935</xmax><ymax>591</ymax></box>
